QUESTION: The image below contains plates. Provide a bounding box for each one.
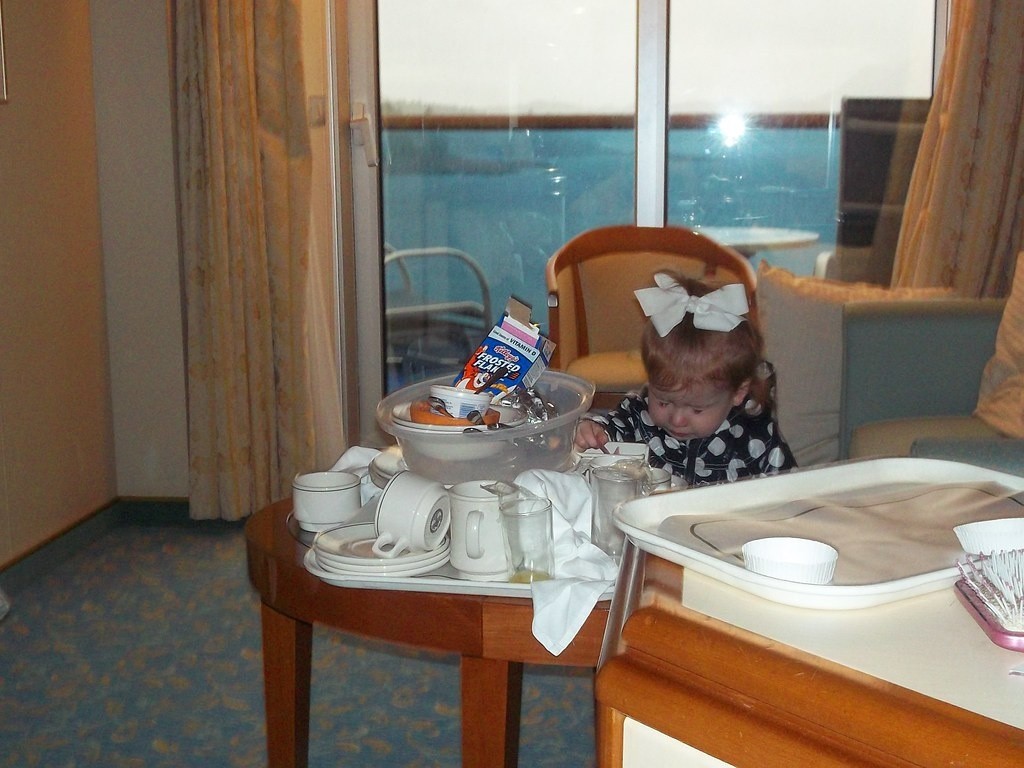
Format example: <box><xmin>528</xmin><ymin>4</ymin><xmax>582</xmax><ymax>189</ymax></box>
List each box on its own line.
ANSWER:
<box><xmin>373</xmin><ymin>449</ymin><xmax>591</xmax><ymax>482</ymax></box>
<box><xmin>314</xmin><ymin>522</ymin><xmax>453</xmax><ymax>577</ymax></box>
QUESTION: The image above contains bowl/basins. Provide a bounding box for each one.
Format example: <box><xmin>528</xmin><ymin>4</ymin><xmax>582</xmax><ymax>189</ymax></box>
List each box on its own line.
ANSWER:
<box><xmin>376</xmin><ymin>368</ymin><xmax>596</xmax><ymax>483</ymax></box>
<box><xmin>391</xmin><ymin>404</ymin><xmax>529</xmax><ymax>460</ymax></box>
<box><xmin>430</xmin><ymin>383</ymin><xmax>494</xmax><ymax>418</ymax></box>
<box><xmin>741</xmin><ymin>537</ymin><xmax>838</xmax><ymax>586</ymax></box>
<box><xmin>953</xmin><ymin>518</ymin><xmax>1024</xmax><ymax>556</ymax></box>
<box><xmin>409</xmin><ymin>399</ymin><xmax>500</xmax><ymax>425</ymax></box>
<box><xmin>578</xmin><ymin>440</ymin><xmax>648</xmax><ymax>466</ymax></box>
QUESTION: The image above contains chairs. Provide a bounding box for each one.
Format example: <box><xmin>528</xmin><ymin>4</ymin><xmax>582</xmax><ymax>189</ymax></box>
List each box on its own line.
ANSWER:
<box><xmin>547</xmin><ymin>225</ymin><xmax>757</xmax><ymax>410</ymax></box>
<box><xmin>815</xmin><ymin>96</ymin><xmax>931</xmax><ymax>283</ymax></box>
<box><xmin>382</xmin><ymin>242</ymin><xmax>491</xmax><ymax>393</ymax></box>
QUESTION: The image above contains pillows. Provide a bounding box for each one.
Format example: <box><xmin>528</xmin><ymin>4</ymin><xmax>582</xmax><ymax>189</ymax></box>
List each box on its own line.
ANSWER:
<box><xmin>978</xmin><ymin>254</ymin><xmax>1024</xmax><ymax>446</ymax></box>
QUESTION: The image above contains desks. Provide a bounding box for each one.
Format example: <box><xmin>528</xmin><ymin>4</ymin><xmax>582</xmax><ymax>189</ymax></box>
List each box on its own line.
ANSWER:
<box><xmin>670</xmin><ymin>222</ymin><xmax>817</xmax><ymax>258</ymax></box>
<box><xmin>245</xmin><ymin>493</ymin><xmax>613</xmax><ymax>768</ymax></box>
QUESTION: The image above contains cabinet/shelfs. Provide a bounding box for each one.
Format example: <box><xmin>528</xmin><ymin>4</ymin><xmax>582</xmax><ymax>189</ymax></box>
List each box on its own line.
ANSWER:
<box><xmin>595</xmin><ymin>611</ymin><xmax>1022</xmax><ymax>768</ymax></box>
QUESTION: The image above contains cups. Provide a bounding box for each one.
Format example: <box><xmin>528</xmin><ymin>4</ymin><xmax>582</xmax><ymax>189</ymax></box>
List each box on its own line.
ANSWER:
<box><xmin>647</xmin><ymin>468</ymin><xmax>672</xmax><ymax>491</ymax></box>
<box><xmin>591</xmin><ymin>465</ymin><xmax>646</xmax><ymax>567</ymax></box>
<box><xmin>500</xmin><ymin>499</ymin><xmax>556</xmax><ymax>581</ymax></box>
<box><xmin>450</xmin><ymin>481</ymin><xmax>522</xmax><ymax>584</ymax></box>
<box><xmin>292</xmin><ymin>471</ymin><xmax>363</xmax><ymax>532</ymax></box>
<box><xmin>371</xmin><ymin>470</ymin><xmax>452</xmax><ymax>557</ymax></box>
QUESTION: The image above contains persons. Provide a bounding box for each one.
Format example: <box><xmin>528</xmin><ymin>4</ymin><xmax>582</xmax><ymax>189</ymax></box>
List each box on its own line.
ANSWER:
<box><xmin>572</xmin><ymin>270</ymin><xmax>800</xmax><ymax>487</ymax></box>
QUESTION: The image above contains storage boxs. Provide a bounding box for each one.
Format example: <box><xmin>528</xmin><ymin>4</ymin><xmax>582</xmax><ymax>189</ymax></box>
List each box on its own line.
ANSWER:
<box><xmin>429</xmin><ymin>385</ymin><xmax>492</xmax><ymax>419</ymax></box>
<box><xmin>450</xmin><ymin>297</ymin><xmax>556</xmax><ymax>407</ymax></box>
